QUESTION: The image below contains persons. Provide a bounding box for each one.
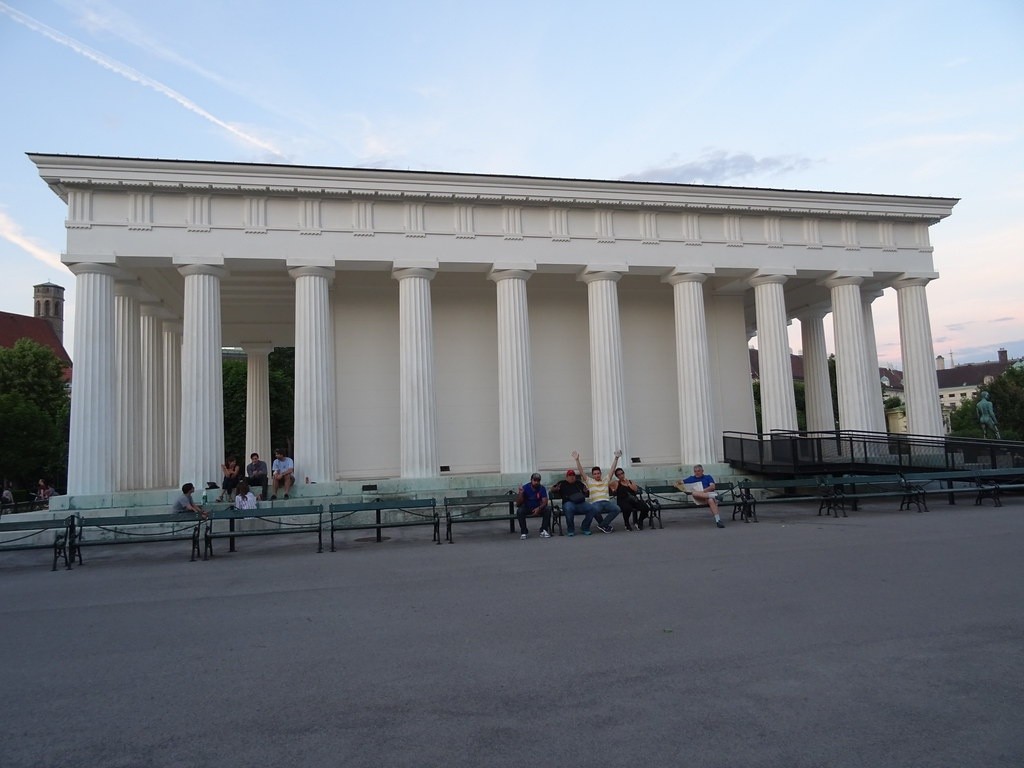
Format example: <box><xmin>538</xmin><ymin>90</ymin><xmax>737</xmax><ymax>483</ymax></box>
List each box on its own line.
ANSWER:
<box><xmin>2</xmin><ymin>486</ymin><xmax>13</xmax><ymax>515</ymax></box>
<box><xmin>270</xmin><ymin>448</ymin><xmax>295</xmax><ymax>500</ymax></box>
<box><xmin>516</xmin><ymin>450</ymin><xmax>648</xmax><ymax>540</ymax></box>
<box><xmin>976</xmin><ymin>391</ymin><xmax>1001</xmax><ymax>440</ymax></box>
<box><xmin>215</xmin><ymin>453</ymin><xmax>268</xmax><ymax>520</ymax></box>
<box><xmin>34</xmin><ymin>478</ymin><xmax>59</xmax><ymax>510</ymax></box>
<box><xmin>673</xmin><ymin>464</ymin><xmax>725</xmax><ymax>528</ymax></box>
<box><xmin>171</xmin><ymin>482</ymin><xmax>209</xmax><ymax>521</ymax></box>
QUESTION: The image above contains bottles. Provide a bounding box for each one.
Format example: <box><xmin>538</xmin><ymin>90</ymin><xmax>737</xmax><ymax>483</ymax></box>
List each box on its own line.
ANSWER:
<box><xmin>202</xmin><ymin>490</ymin><xmax>207</xmax><ymax>503</ymax></box>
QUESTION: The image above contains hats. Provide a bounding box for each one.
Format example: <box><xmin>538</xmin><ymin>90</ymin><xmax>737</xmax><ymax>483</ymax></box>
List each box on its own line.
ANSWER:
<box><xmin>566</xmin><ymin>468</ymin><xmax>575</xmax><ymax>476</ymax></box>
<box><xmin>532</xmin><ymin>474</ymin><xmax>541</xmax><ymax>480</ymax></box>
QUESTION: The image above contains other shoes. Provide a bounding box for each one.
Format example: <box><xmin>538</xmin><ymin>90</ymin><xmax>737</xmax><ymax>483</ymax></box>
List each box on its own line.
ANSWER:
<box><xmin>283</xmin><ymin>493</ymin><xmax>290</xmax><ymax>500</ymax></box>
<box><xmin>216</xmin><ymin>496</ymin><xmax>224</xmax><ymax>502</ymax></box>
<box><xmin>597</xmin><ymin>526</ymin><xmax>612</xmax><ymax>534</ymax></box>
<box><xmin>228</xmin><ymin>498</ymin><xmax>234</xmax><ymax>503</ymax></box>
<box><xmin>540</xmin><ymin>530</ymin><xmax>550</xmax><ymax>538</ymax></box>
<box><xmin>635</xmin><ymin>523</ymin><xmax>641</xmax><ymax>531</ymax></box>
<box><xmin>708</xmin><ymin>492</ymin><xmax>719</xmax><ymax>499</ymax></box>
<box><xmin>607</xmin><ymin>524</ymin><xmax>614</xmax><ymax>530</ymax></box>
<box><xmin>579</xmin><ymin>528</ymin><xmax>591</xmax><ymax>535</ymax></box>
<box><xmin>269</xmin><ymin>494</ymin><xmax>277</xmax><ymax>501</ymax></box>
<box><xmin>566</xmin><ymin>530</ymin><xmax>574</xmax><ymax>537</ymax></box>
<box><xmin>520</xmin><ymin>534</ymin><xmax>527</xmax><ymax>540</ymax></box>
<box><xmin>624</xmin><ymin>526</ymin><xmax>631</xmax><ymax>532</ymax></box>
<box><xmin>717</xmin><ymin>520</ymin><xmax>725</xmax><ymax>528</ymax></box>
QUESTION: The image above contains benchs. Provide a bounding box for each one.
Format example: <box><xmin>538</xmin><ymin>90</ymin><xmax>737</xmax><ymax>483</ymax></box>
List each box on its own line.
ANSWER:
<box><xmin>329</xmin><ymin>497</ymin><xmax>443</xmax><ymax>553</ymax></box>
<box><xmin>548</xmin><ymin>487</ymin><xmax>657</xmax><ymax>536</ymax></box>
<box><xmin>444</xmin><ymin>492</ymin><xmax>554</xmax><ymax>544</ymax></box>
<box><xmin>645</xmin><ymin>481</ymin><xmax>751</xmax><ymax>529</ymax></box>
<box><xmin>975</xmin><ymin>467</ymin><xmax>1024</xmax><ymax>507</ymax></box>
<box><xmin>65</xmin><ymin>510</ymin><xmax>204</xmax><ymax>570</ymax></box>
<box><xmin>201</xmin><ymin>503</ymin><xmax>324</xmax><ymax>562</ymax></box>
<box><xmin>900</xmin><ymin>469</ymin><xmax>999</xmax><ymax>512</ymax></box>
<box><xmin>821</xmin><ymin>473</ymin><xmax>923</xmax><ymax>517</ymax></box>
<box><xmin>736</xmin><ymin>477</ymin><xmax>839</xmax><ymax>523</ymax></box>
<box><xmin>0</xmin><ymin>516</ymin><xmax>69</xmax><ymax>571</ymax></box>
